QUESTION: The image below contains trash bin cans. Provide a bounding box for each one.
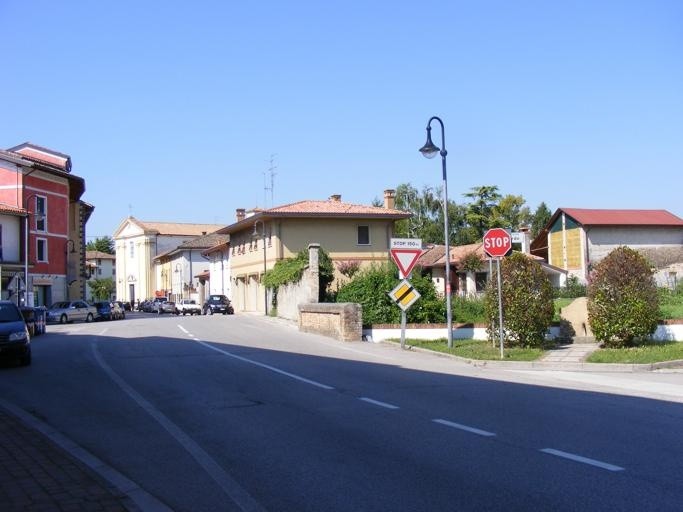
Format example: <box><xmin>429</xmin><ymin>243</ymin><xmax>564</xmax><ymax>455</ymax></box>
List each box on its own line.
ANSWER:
<box><xmin>34</xmin><ymin>305</ymin><xmax>46</xmax><ymax>335</ymax></box>
<box><xmin>21</xmin><ymin>306</ymin><xmax>35</xmax><ymax>337</ymax></box>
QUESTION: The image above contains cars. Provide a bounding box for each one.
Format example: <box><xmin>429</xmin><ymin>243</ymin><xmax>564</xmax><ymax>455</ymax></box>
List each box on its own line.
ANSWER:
<box><xmin>91</xmin><ymin>302</ymin><xmax>131</xmax><ymax>321</ymax></box>
<box><xmin>0</xmin><ymin>300</ymin><xmax>31</xmax><ymax>368</ymax></box>
<box><xmin>141</xmin><ymin>298</ymin><xmax>201</xmax><ymax>316</ymax></box>
<box><xmin>202</xmin><ymin>295</ymin><xmax>234</xmax><ymax>315</ymax></box>
<box><xmin>46</xmin><ymin>300</ymin><xmax>98</xmax><ymax>324</ymax></box>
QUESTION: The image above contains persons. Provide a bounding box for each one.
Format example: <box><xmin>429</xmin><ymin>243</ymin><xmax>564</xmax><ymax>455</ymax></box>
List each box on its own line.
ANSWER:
<box><xmin>131</xmin><ymin>297</ymin><xmax>135</xmax><ymax>312</ymax></box>
<box><xmin>137</xmin><ymin>299</ymin><xmax>141</xmax><ymax>312</ymax></box>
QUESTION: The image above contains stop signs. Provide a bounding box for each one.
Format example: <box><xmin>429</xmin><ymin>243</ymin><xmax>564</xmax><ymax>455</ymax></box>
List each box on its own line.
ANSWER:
<box><xmin>483</xmin><ymin>228</ymin><xmax>512</xmax><ymax>258</ymax></box>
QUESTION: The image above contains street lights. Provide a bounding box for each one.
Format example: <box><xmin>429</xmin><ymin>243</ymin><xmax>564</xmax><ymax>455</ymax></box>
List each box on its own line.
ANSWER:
<box><xmin>419</xmin><ymin>117</ymin><xmax>453</xmax><ymax>348</ymax></box>
<box><xmin>65</xmin><ymin>240</ymin><xmax>78</xmax><ymax>301</ymax></box>
<box><xmin>252</xmin><ymin>220</ymin><xmax>267</xmax><ymax>315</ymax></box>
<box><xmin>25</xmin><ymin>195</ymin><xmax>46</xmax><ymax>307</ymax></box>
<box><xmin>175</xmin><ymin>263</ymin><xmax>182</xmax><ymax>299</ymax></box>
